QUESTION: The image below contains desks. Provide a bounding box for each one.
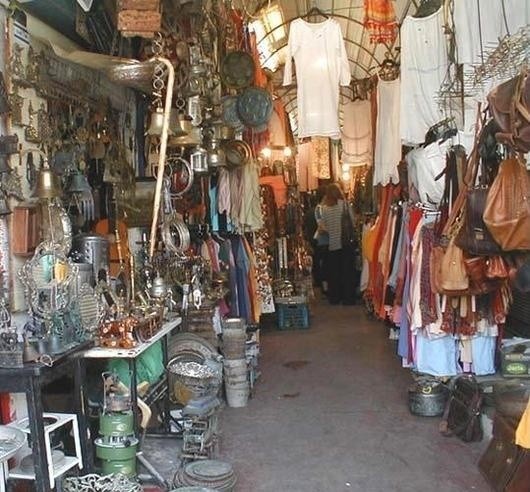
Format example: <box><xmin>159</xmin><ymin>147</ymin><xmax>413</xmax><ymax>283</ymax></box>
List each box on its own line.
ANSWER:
<box><xmin>170</xmin><ymin>287</ymin><xmax>231</xmax><ymax>359</ymax></box>
<box><xmin>0</xmin><ymin>339</ymin><xmax>96</xmax><ymax>492</ymax></box>
<box><xmin>82</xmin><ymin>313</ymin><xmax>182</xmax><ymax>490</ymax></box>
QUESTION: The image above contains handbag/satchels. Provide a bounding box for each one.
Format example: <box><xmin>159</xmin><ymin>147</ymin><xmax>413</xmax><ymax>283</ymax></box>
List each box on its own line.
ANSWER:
<box><xmin>341</xmin><ymin>200</ymin><xmax>358</xmax><ymax>250</ymax></box>
<box><xmin>441</xmin><ymin>375</ymin><xmax>482</xmax><ymax>446</ymax></box>
<box><xmin>486</xmin><ymin>73</ymin><xmax>530</xmax><ymax>153</ymax></box>
<box><xmin>430</xmin><ymin>144</ymin><xmax>530</xmax><ymax>292</ymax></box>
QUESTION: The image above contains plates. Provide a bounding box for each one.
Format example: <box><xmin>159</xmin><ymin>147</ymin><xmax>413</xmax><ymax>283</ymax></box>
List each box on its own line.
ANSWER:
<box><xmin>178</xmin><ymin>467</ymin><xmax>231</xmax><ymax>491</ymax></box>
<box><xmin>182</xmin><ymin>468</ymin><xmax>235</xmax><ymax>488</ymax></box>
<box><xmin>173</xmin><ymin>468</ymin><xmax>237</xmax><ymax>491</ymax></box>
<box><xmin>185</xmin><ymin>459</ymin><xmax>233</xmax><ymax>482</ymax></box>
<box><xmin>193</xmin><ymin>462</ymin><xmax>232</xmax><ymax>476</ymax></box>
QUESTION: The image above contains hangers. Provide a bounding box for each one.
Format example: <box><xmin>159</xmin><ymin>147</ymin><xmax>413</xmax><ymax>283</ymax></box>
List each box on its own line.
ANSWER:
<box><xmin>399</xmin><ymin>199</ymin><xmax>444</xmax><ymax>222</ymax></box>
<box><xmin>301</xmin><ymin>7</ymin><xmax>328</xmax><ymax>24</ymax></box>
<box><xmin>412</xmin><ymin>0</ymin><xmax>441</xmax><ymax>18</ymax></box>
<box><xmin>409</xmin><ymin>118</ymin><xmax>460</xmax><ymax>149</ymax></box>
<box><xmin>437</xmin><ymin>149</ymin><xmax>446</xmax><ymax>156</ymax></box>
<box><xmin>373</xmin><ymin>52</ymin><xmax>400</xmax><ymax>78</ymax></box>
<box><xmin>351</xmin><ymin>93</ymin><xmax>365</xmax><ymax>102</ymax></box>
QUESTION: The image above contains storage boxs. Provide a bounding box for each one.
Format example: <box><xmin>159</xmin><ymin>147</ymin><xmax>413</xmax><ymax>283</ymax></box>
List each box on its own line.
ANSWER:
<box><xmin>279</xmin><ymin>305</ymin><xmax>311</xmax><ymax>330</ymax></box>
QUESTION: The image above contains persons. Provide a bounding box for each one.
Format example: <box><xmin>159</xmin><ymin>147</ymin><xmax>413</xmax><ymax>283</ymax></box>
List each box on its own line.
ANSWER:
<box><xmin>313</xmin><ymin>185</ymin><xmax>330</xmax><ymax>295</ymax></box>
<box><xmin>322</xmin><ymin>185</ymin><xmax>355</xmax><ymax>305</ymax></box>
